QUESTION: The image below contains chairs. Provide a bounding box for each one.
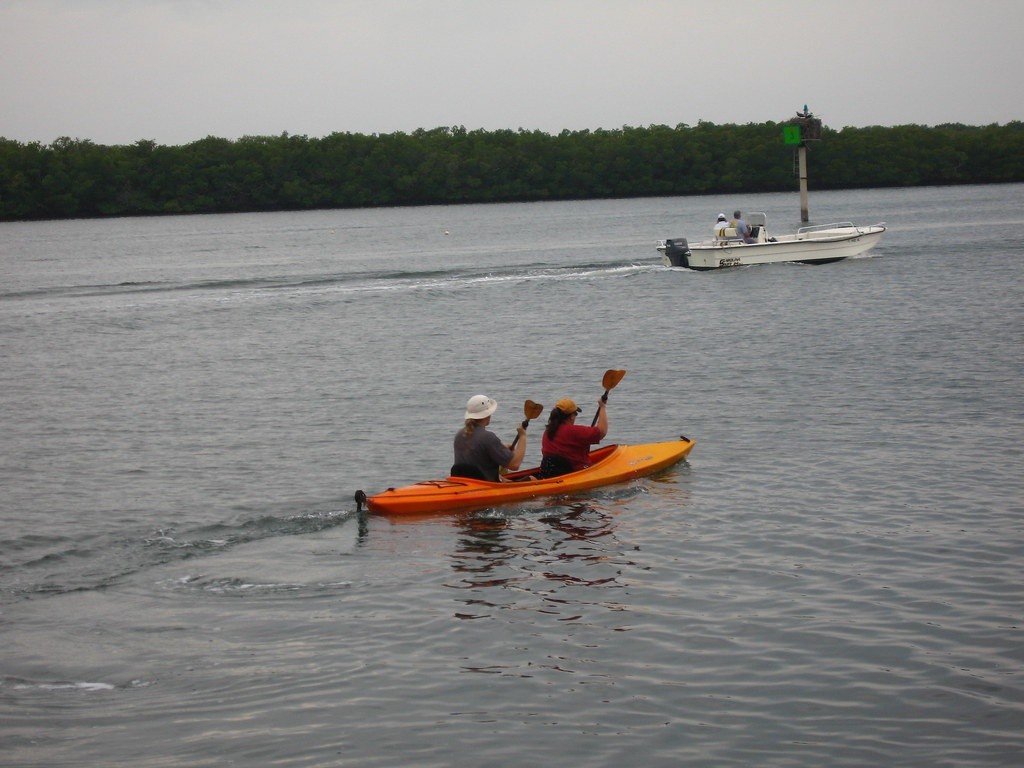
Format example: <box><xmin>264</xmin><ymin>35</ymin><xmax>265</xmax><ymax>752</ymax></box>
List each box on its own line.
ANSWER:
<box><xmin>450</xmin><ymin>462</ymin><xmax>487</xmax><ymax>479</ymax></box>
<box><xmin>540</xmin><ymin>456</ymin><xmax>574</xmax><ymax>477</ymax></box>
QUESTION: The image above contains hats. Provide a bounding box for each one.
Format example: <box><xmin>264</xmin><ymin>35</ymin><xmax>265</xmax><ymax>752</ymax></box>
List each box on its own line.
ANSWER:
<box><xmin>556</xmin><ymin>397</ymin><xmax>582</xmax><ymax>415</ymax></box>
<box><xmin>464</xmin><ymin>395</ymin><xmax>498</xmax><ymax>419</ymax></box>
<box><xmin>718</xmin><ymin>213</ymin><xmax>725</xmax><ymax>218</ymax></box>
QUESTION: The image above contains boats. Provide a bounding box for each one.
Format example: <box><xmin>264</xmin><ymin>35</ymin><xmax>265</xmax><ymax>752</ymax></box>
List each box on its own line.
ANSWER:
<box><xmin>352</xmin><ymin>433</ymin><xmax>697</xmax><ymax>516</ymax></box>
<box><xmin>657</xmin><ymin>219</ymin><xmax>889</xmax><ymax>271</ymax></box>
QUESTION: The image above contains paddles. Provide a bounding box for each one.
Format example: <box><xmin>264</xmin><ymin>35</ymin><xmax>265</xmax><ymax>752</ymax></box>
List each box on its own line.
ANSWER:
<box><xmin>500</xmin><ymin>400</ymin><xmax>543</xmax><ymax>470</ymax></box>
<box><xmin>585</xmin><ymin>370</ymin><xmax>626</xmax><ymax>452</ymax></box>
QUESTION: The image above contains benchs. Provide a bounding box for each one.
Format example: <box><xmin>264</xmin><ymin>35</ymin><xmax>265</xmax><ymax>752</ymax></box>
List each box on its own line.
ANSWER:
<box><xmin>714</xmin><ymin>228</ymin><xmax>744</xmax><ymax>245</ymax></box>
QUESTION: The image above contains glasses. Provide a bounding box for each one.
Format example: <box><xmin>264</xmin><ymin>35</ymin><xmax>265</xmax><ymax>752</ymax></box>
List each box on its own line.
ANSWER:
<box><xmin>574</xmin><ymin>412</ymin><xmax>579</xmax><ymax>416</ymax></box>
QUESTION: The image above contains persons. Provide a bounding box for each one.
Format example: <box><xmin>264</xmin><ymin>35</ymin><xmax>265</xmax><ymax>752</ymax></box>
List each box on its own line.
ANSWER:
<box><xmin>541</xmin><ymin>396</ymin><xmax>608</xmax><ymax>472</ymax></box>
<box><xmin>453</xmin><ymin>393</ymin><xmax>526</xmax><ymax>483</ymax></box>
<box><xmin>713</xmin><ymin>210</ymin><xmax>752</xmax><ymax>240</ymax></box>
